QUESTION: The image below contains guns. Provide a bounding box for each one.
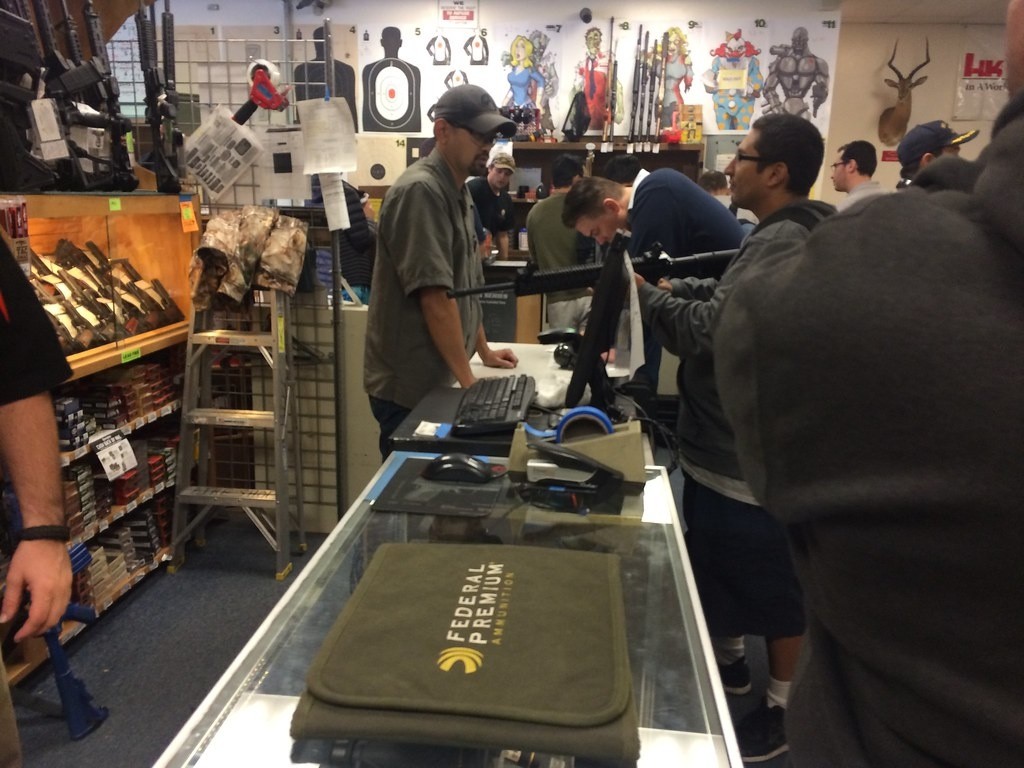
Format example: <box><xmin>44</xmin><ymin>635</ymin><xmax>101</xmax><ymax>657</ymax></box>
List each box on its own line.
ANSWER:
<box><xmin>626</xmin><ymin>23</ymin><xmax>670</xmax><ymax>143</ymax></box>
<box><xmin>601</xmin><ymin>16</ymin><xmax>618</xmax><ymax>143</ymax></box>
<box><xmin>0</xmin><ymin>1</ymin><xmax>141</xmax><ymax>192</ymax></box>
<box><xmin>132</xmin><ymin>1</ymin><xmax>185</xmax><ymax>195</ymax></box>
<box><xmin>445</xmin><ymin>240</ymin><xmax>743</xmax><ymax>303</ymax></box>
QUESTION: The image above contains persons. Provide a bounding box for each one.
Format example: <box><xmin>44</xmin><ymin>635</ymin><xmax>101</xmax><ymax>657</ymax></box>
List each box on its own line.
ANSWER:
<box><xmin>832</xmin><ymin>141</ymin><xmax>886</xmax><ymax>212</ymax></box>
<box><xmin>700</xmin><ymin>170</ymin><xmax>760</xmax><ymax>232</ymax></box>
<box><xmin>363</xmin><ymin>83</ymin><xmax>517</xmax><ymax>464</ymax></box>
<box><xmin>601</xmin><ymin>154</ymin><xmax>642</xmax><ymax>188</ymax></box>
<box><xmin>562</xmin><ymin>168</ymin><xmax>746</xmax><ymax>363</ymax></box>
<box><xmin>1</xmin><ymin>235</ymin><xmax>74</xmax><ymax>643</ymax></box>
<box><xmin>715</xmin><ymin>0</ymin><xmax>1024</xmax><ymax>767</ymax></box>
<box><xmin>898</xmin><ymin>121</ymin><xmax>978</xmax><ymax>181</ymax></box>
<box><xmin>526</xmin><ymin>155</ymin><xmax>596</xmax><ymax>334</ymax></box>
<box><xmin>632</xmin><ymin>115</ymin><xmax>837</xmax><ymax>762</ymax></box>
<box><xmin>466</xmin><ymin>153</ymin><xmax>516</xmax><ymax>260</ymax></box>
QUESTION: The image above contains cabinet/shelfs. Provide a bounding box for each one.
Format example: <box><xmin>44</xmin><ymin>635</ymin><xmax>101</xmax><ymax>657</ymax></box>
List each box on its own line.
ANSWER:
<box><xmin>484</xmin><ymin>135</ymin><xmax>704</xmax><ymax>262</ymax></box>
<box><xmin>0</xmin><ymin>194</ymin><xmax>202</xmax><ymax>689</ymax></box>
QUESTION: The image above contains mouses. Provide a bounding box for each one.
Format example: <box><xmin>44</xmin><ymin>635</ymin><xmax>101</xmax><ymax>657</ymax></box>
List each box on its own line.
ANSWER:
<box><xmin>422</xmin><ymin>453</ymin><xmax>493</xmax><ymax>484</ymax></box>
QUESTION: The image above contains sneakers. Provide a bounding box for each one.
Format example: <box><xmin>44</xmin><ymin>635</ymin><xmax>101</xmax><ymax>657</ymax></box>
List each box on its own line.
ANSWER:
<box><xmin>736</xmin><ymin>696</ymin><xmax>790</xmax><ymax>762</ymax></box>
<box><xmin>718</xmin><ymin>653</ymin><xmax>752</xmax><ymax>693</ymax></box>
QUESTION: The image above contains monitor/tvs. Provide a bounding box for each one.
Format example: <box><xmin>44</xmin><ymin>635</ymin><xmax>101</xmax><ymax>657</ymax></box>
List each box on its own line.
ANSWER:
<box><xmin>566</xmin><ymin>228</ymin><xmax>629</xmax><ymax>423</ymax></box>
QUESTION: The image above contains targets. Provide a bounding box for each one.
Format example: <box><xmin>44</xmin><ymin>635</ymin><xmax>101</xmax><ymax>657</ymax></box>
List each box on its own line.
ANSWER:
<box><xmin>367</xmin><ymin>57</ymin><xmax>416</xmax><ymax>130</ymax></box>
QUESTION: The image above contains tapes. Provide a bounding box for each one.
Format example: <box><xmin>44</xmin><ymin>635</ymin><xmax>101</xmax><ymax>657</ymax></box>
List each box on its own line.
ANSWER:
<box><xmin>522</xmin><ymin>406</ymin><xmax>613</xmax><ymax>444</ymax></box>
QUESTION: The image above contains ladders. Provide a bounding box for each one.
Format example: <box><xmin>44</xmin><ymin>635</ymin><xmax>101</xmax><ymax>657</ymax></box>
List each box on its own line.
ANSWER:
<box><xmin>173</xmin><ymin>289</ymin><xmax>306</xmax><ymax>579</ymax></box>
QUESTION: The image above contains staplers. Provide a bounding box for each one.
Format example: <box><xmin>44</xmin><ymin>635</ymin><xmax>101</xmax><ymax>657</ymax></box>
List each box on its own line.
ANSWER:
<box><xmin>520</xmin><ymin>443</ymin><xmax>625</xmax><ymax>517</ymax></box>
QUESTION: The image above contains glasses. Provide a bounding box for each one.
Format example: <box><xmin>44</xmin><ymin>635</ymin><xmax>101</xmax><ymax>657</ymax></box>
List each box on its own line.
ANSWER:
<box><xmin>830</xmin><ymin>161</ymin><xmax>848</xmax><ymax>171</ymax></box>
<box><xmin>736</xmin><ymin>147</ymin><xmax>774</xmax><ymax>164</ymax></box>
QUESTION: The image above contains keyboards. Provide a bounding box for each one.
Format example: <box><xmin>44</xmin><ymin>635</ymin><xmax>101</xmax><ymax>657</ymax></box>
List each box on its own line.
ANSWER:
<box><xmin>454</xmin><ymin>375</ymin><xmax>536</xmax><ymax>435</ymax></box>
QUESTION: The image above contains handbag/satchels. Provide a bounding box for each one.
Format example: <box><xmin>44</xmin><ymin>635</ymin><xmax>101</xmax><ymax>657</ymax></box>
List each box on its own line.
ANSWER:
<box><xmin>290</xmin><ymin>542</ymin><xmax>640</xmax><ymax>768</ymax></box>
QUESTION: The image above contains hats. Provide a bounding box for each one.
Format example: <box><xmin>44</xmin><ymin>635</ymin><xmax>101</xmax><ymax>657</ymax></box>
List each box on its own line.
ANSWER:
<box><xmin>896</xmin><ymin>119</ymin><xmax>980</xmax><ymax>169</ymax></box>
<box><xmin>435</xmin><ymin>84</ymin><xmax>517</xmax><ymax>139</ymax></box>
<box><xmin>490</xmin><ymin>152</ymin><xmax>515</xmax><ymax>173</ymax></box>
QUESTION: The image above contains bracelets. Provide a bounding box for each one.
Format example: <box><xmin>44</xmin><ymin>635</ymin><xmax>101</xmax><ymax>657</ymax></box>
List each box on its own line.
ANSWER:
<box><xmin>18</xmin><ymin>526</ymin><xmax>70</xmax><ymax>541</ymax></box>
